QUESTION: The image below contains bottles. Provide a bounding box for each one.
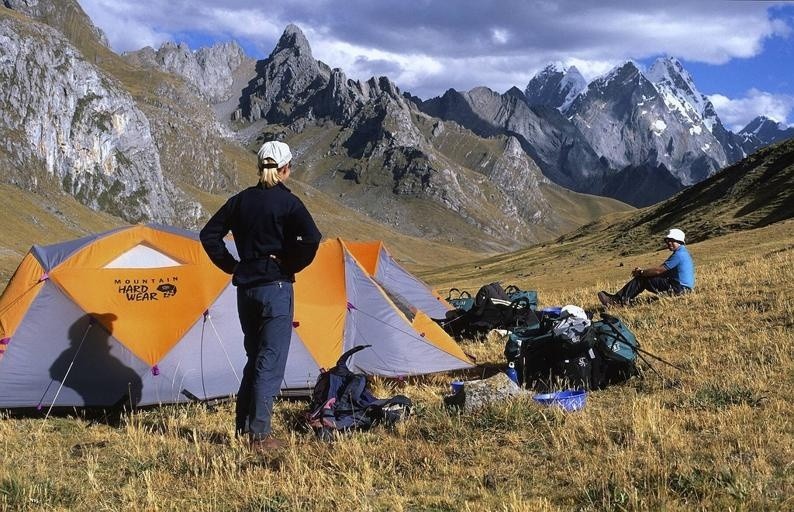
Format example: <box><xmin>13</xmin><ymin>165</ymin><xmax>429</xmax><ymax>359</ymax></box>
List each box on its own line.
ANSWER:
<box><xmin>506</xmin><ymin>361</ymin><xmax>518</xmax><ymax>385</ymax></box>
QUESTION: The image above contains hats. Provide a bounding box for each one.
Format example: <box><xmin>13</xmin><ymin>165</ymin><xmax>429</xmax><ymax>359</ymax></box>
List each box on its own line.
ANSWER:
<box><xmin>664</xmin><ymin>228</ymin><xmax>686</xmax><ymax>244</ymax></box>
<box><xmin>256</xmin><ymin>140</ymin><xmax>293</xmax><ymax>169</ymax></box>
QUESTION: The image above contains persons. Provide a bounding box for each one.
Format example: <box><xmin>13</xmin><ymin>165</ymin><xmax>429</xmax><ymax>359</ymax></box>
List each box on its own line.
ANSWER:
<box><xmin>199</xmin><ymin>142</ymin><xmax>322</xmax><ymax>452</ymax></box>
<box><xmin>599</xmin><ymin>228</ymin><xmax>694</xmax><ymax>308</ymax></box>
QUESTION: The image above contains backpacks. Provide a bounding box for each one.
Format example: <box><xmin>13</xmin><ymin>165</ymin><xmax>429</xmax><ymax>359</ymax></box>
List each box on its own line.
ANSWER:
<box><xmin>306</xmin><ymin>344</ymin><xmax>413</xmax><ymax>443</ymax></box>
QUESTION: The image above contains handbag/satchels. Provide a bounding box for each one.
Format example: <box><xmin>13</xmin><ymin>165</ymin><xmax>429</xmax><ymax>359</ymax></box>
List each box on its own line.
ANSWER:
<box><xmin>446</xmin><ymin>280</ymin><xmax>638</xmax><ymax>396</ymax></box>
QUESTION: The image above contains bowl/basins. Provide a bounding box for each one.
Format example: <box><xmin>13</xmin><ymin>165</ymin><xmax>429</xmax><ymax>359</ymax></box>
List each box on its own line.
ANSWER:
<box><xmin>532</xmin><ymin>391</ymin><xmax>587</xmax><ymax>410</ymax></box>
<box><xmin>541</xmin><ymin>307</ymin><xmax>561</xmax><ymax>315</ymax></box>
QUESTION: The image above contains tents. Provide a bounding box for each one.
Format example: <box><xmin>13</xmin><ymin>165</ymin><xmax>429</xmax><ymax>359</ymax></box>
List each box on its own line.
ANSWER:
<box><xmin>1</xmin><ymin>222</ymin><xmax>324</xmax><ymax>409</ymax></box>
<box><xmin>345</xmin><ymin>241</ymin><xmax>459</xmax><ymax>320</ymax></box>
<box><xmin>288</xmin><ymin>238</ymin><xmax>478</xmax><ymax>379</ymax></box>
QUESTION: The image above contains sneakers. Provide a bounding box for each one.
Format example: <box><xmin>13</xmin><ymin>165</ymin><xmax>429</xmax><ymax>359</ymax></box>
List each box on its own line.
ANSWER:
<box><xmin>597</xmin><ymin>291</ymin><xmax>616</xmax><ymax>306</ymax></box>
<box><xmin>251</xmin><ymin>438</ymin><xmax>282</xmax><ymax>453</ymax></box>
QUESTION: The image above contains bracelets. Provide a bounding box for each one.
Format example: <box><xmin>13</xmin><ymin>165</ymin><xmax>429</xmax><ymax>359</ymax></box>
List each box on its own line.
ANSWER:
<box><xmin>640</xmin><ymin>270</ymin><xmax>644</xmax><ymax>275</ymax></box>
